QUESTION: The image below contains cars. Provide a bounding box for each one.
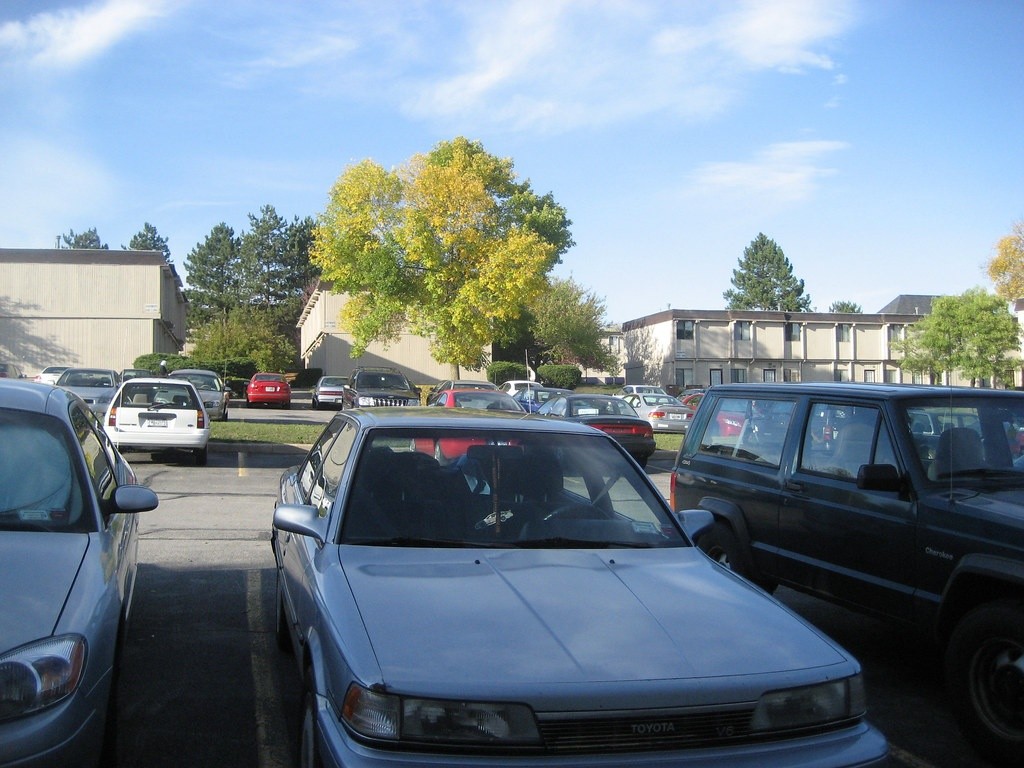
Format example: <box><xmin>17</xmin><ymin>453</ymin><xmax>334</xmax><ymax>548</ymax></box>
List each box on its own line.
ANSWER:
<box><xmin>616</xmin><ymin>385</ymin><xmax>668</xmax><ymax>396</ymax></box>
<box><xmin>0</xmin><ymin>376</ymin><xmax>159</xmax><ymax>768</ymax></box>
<box><xmin>243</xmin><ymin>373</ymin><xmax>293</xmax><ymax>410</ymax></box>
<box><xmin>102</xmin><ymin>377</ymin><xmax>214</xmax><ymax>467</ymax></box>
<box><xmin>429</xmin><ymin>379</ymin><xmax>501</xmax><ymax>392</ymax></box>
<box><xmin>612</xmin><ymin>393</ymin><xmax>697</xmax><ymax>435</ymax></box>
<box><xmin>408</xmin><ymin>388</ymin><xmax>531</xmax><ymax>468</ymax></box>
<box><xmin>118</xmin><ymin>369</ymin><xmax>159</xmax><ymax>390</ymax></box>
<box><xmin>499</xmin><ymin>380</ymin><xmax>544</xmax><ymax>397</ymax></box>
<box><xmin>0</xmin><ymin>363</ymin><xmax>28</xmax><ymax>379</ymax></box>
<box><xmin>527</xmin><ymin>394</ymin><xmax>656</xmax><ymax>471</ymax></box>
<box><xmin>272</xmin><ymin>406</ymin><xmax>895</xmax><ymax>768</ymax></box>
<box><xmin>166</xmin><ymin>369</ymin><xmax>231</xmax><ymax>423</ymax></box>
<box><xmin>675</xmin><ymin>387</ymin><xmax>1024</xmax><ymax>480</ymax></box>
<box><xmin>33</xmin><ymin>365</ymin><xmax>71</xmax><ymax>387</ymax></box>
<box><xmin>47</xmin><ymin>367</ymin><xmax>123</xmax><ymax>417</ymax></box>
<box><xmin>512</xmin><ymin>386</ymin><xmax>577</xmax><ymax>413</ymax></box>
<box><xmin>312</xmin><ymin>375</ymin><xmax>351</xmax><ymax>412</ymax></box>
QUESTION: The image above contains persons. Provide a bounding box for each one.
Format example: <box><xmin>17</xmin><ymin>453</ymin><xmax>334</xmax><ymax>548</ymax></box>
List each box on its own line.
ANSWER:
<box><xmin>160</xmin><ymin>361</ymin><xmax>168</xmax><ymax>377</ymax></box>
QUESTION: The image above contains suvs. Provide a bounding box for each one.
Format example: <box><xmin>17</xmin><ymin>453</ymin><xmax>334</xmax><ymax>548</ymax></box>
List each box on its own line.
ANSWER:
<box><xmin>341</xmin><ymin>366</ymin><xmax>423</xmax><ymax>411</ymax></box>
<box><xmin>670</xmin><ymin>380</ymin><xmax>1024</xmax><ymax>768</ymax></box>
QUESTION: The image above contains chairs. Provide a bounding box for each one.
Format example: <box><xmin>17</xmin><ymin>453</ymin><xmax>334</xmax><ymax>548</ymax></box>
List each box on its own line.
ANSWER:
<box><xmin>172</xmin><ymin>395</ymin><xmax>189</xmax><ymax>405</ymax></box>
<box><xmin>347</xmin><ymin>452</ymin><xmax>468</xmax><ymax>542</ymax></box>
<box><xmin>928</xmin><ymin>427</ymin><xmax>987</xmax><ymax>482</ymax></box>
<box><xmin>467</xmin><ymin>445</ymin><xmax>528</xmax><ymax>497</ymax></box>
<box><xmin>100</xmin><ymin>377</ymin><xmax>110</xmax><ymax>386</ymax></box>
<box><xmin>132</xmin><ymin>393</ymin><xmax>148</xmax><ymax>403</ymax></box>
<box><xmin>204</xmin><ymin>380</ymin><xmax>216</xmax><ymax>388</ymax></box>
<box><xmin>71</xmin><ymin>375</ymin><xmax>82</xmax><ymax>383</ymax></box>
<box><xmin>826</xmin><ymin>423</ymin><xmax>883</xmax><ymax>478</ymax></box>
